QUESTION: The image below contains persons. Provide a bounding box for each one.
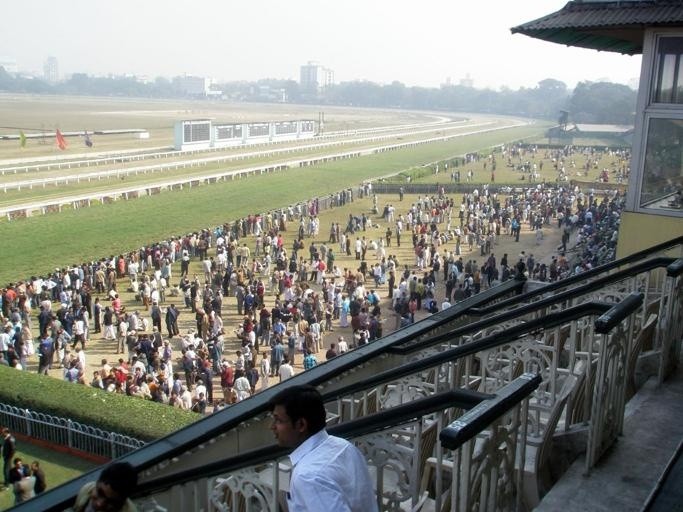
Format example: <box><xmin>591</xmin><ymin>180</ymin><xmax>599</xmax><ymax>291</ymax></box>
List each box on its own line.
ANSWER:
<box><xmin>1</xmin><ymin>254</ymin><xmax>294</xmax><ymax>416</ymax></box>
<box><xmin>0</xmin><ymin>426</ymin><xmax>16</xmax><ymax>488</ymax></box>
<box><xmin>69</xmin><ymin>461</ymin><xmax>140</xmax><ymax>512</ymax></box>
<box><xmin>30</xmin><ymin>460</ymin><xmax>46</xmax><ymax>496</ymax></box>
<box><xmin>7</xmin><ymin>458</ymin><xmax>30</xmax><ymax>485</ymax></box>
<box><xmin>140</xmin><ymin>197</ymin><xmax>481</xmax><ymax>372</ymax></box>
<box><xmin>265</xmin><ymin>383</ymin><xmax>379</xmax><ymax>511</ymax></box>
<box><xmin>11</xmin><ymin>466</ymin><xmax>36</xmax><ymax>505</ymax></box>
<box><xmin>330</xmin><ymin>176</ymin><xmax>626</xmax><ymax>288</ymax></box>
<box><xmin>438</xmin><ymin>142</ymin><xmax>683</xmax><ymax>195</ymax></box>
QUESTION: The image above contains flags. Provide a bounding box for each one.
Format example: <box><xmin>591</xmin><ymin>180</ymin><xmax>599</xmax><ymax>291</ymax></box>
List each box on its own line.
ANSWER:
<box><xmin>84</xmin><ymin>129</ymin><xmax>92</xmax><ymax>149</ymax></box>
<box><xmin>19</xmin><ymin>130</ymin><xmax>29</xmax><ymax>151</ymax></box>
<box><xmin>56</xmin><ymin>128</ymin><xmax>67</xmax><ymax>151</ymax></box>
